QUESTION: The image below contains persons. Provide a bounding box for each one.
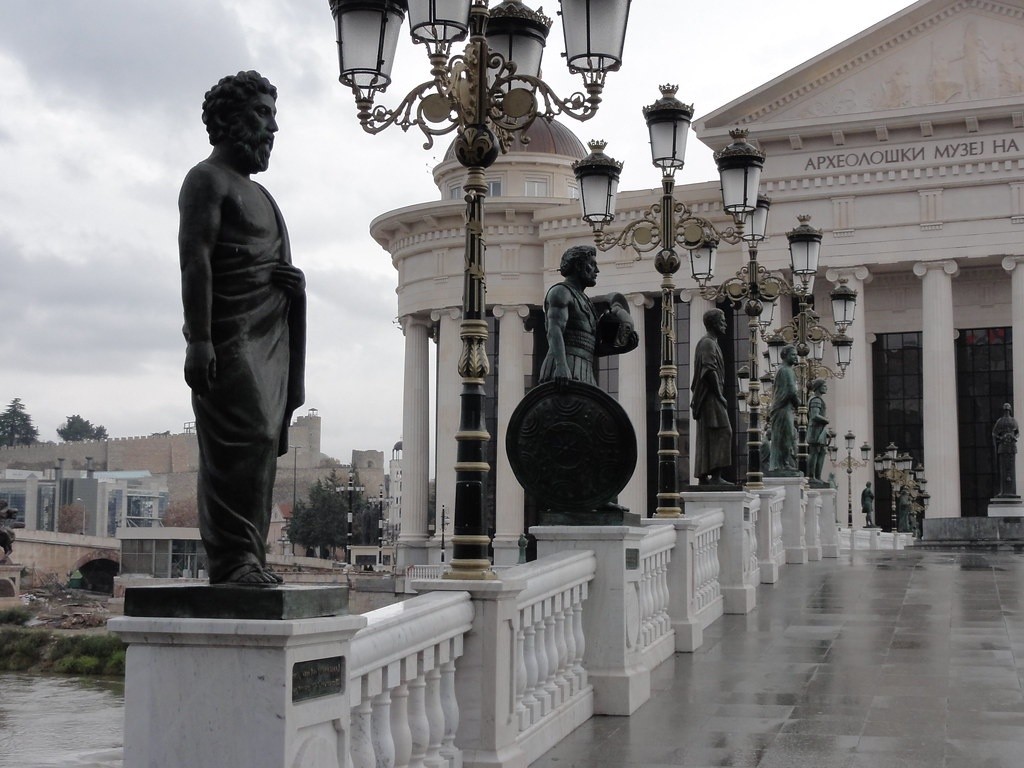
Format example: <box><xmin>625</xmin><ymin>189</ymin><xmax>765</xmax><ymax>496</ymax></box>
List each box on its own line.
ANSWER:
<box><xmin>806</xmin><ymin>379</ymin><xmax>830</xmax><ymax>484</ymax></box>
<box><xmin>898</xmin><ymin>491</ymin><xmax>913</xmax><ymax>532</ymax></box>
<box><xmin>993</xmin><ymin>404</ymin><xmax>1020</xmax><ymax>495</ymax></box>
<box><xmin>691</xmin><ymin>308</ymin><xmax>734</xmax><ymax>485</ymax></box>
<box><xmin>861</xmin><ymin>481</ymin><xmax>874</xmax><ymax>528</ymax></box>
<box><xmin>768</xmin><ymin>345</ymin><xmax>801</xmax><ymax>473</ymax></box>
<box><xmin>539</xmin><ymin>245</ymin><xmax>639</xmax><ymax>514</ymax></box>
<box><xmin>828</xmin><ymin>472</ymin><xmax>839</xmax><ymax>524</ymax></box>
<box><xmin>179</xmin><ymin>71</ymin><xmax>306</xmax><ymax>588</ymax></box>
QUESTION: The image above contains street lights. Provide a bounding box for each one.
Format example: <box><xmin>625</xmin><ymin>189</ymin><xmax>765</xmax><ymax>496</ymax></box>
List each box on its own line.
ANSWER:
<box><xmin>441</xmin><ymin>504</ymin><xmax>450</xmax><ymax>565</ymax></box>
<box><xmin>335</xmin><ymin>469</ymin><xmax>355</xmax><ymax>572</ymax></box>
<box><xmin>689</xmin><ymin>190</ymin><xmax>859</xmax><ymax>490</ymax></box>
<box><xmin>572</xmin><ymin>82</ymin><xmax>766</xmax><ymax>519</ymax></box>
<box><xmin>875</xmin><ymin>442</ymin><xmax>931</xmax><ymax>533</ymax></box>
<box><xmin>329</xmin><ymin>0</ymin><xmax>632</xmax><ymax>580</ymax></box>
<box><xmin>827</xmin><ymin>428</ymin><xmax>871</xmax><ymax>528</ymax></box>
<box><xmin>289</xmin><ymin>444</ymin><xmax>302</xmax><ymax>556</ymax></box>
<box><xmin>367</xmin><ymin>482</ymin><xmax>393</xmax><ymax>572</ymax></box>
